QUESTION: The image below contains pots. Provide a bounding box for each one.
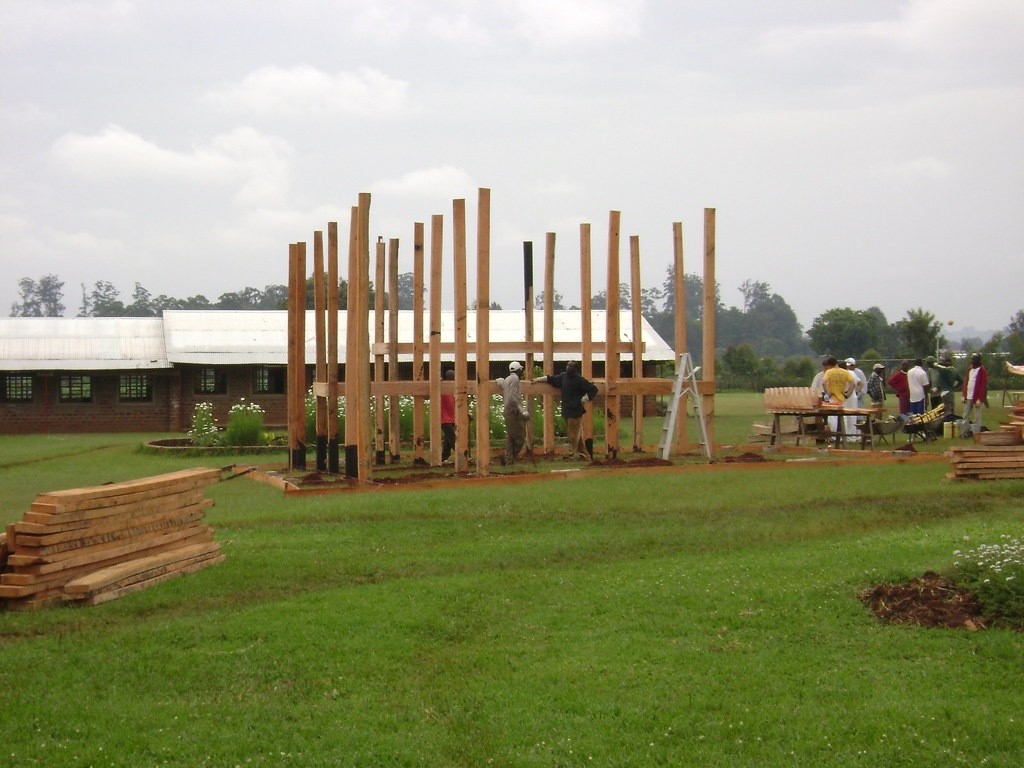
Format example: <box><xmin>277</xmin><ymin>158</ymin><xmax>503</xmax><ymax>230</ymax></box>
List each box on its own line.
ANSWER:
<box><xmin>854</xmin><ymin>417</ymin><xmax>905</xmax><ymax>435</ymax></box>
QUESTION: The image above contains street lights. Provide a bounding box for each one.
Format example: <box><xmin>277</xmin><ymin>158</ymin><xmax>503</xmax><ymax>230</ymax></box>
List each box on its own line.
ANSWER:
<box><xmin>937</xmin><ymin>320</ymin><xmax>954</xmax><ymax>364</ymax></box>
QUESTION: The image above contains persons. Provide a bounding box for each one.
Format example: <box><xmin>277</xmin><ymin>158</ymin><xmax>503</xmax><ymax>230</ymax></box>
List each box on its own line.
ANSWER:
<box><xmin>867</xmin><ymin>363</ymin><xmax>886</xmax><ymax>420</ymax></box>
<box><xmin>440</xmin><ymin>370</ymin><xmax>475</xmax><ymax>464</ymax></box>
<box><xmin>925</xmin><ymin>357</ymin><xmax>943</xmax><ymax>411</ymax></box>
<box><xmin>907</xmin><ymin>358</ymin><xmax>929</xmax><ymax>415</ymax></box>
<box><xmin>959</xmin><ymin>353</ymin><xmax>988</xmax><ymax>439</ymax></box>
<box><xmin>530</xmin><ymin>359</ymin><xmax>598</xmax><ymax>451</ymax></box>
<box><xmin>888</xmin><ymin>360</ymin><xmax>911</xmax><ymax>420</ymax></box>
<box><xmin>496</xmin><ymin>362</ymin><xmax>530</xmax><ymax>465</ymax></box>
<box><xmin>931</xmin><ymin>353</ymin><xmax>964</xmax><ymax>417</ymax></box>
<box><xmin>811</xmin><ymin>357</ymin><xmax>867</xmax><ymax>443</ymax></box>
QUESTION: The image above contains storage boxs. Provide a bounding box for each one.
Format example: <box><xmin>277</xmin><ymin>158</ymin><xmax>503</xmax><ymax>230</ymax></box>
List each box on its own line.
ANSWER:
<box><xmin>943</xmin><ymin>422</ymin><xmax>959</xmax><ymax>440</ymax></box>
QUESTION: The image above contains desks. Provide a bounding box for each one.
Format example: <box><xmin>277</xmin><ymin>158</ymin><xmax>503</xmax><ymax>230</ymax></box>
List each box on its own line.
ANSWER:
<box><xmin>764</xmin><ymin>406</ymin><xmax>879</xmax><ymax>451</ymax></box>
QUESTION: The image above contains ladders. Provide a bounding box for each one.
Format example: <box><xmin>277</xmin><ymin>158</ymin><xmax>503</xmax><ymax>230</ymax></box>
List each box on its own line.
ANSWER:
<box><xmin>655</xmin><ymin>353</ymin><xmax>712</xmax><ymax>461</ymax></box>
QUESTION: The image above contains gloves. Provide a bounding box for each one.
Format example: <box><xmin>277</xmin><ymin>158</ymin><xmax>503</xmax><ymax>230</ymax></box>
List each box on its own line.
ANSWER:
<box><xmin>976</xmin><ymin>399</ymin><xmax>981</xmax><ymax>408</ymax></box>
<box><xmin>522</xmin><ymin>411</ymin><xmax>530</xmax><ymax>422</ymax></box>
<box><xmin>581</xmin><ymin>394</ymin><xmax>589</xmax><ymax>405</ymax></box>
<box><xmin>961</xmin><ymin>397</ymin><xmax>966</xmax><ymax>403</ymax></box>
<box><xmin>530</xmin><ymin>375</ymin><xmax>548</xmax><ymax>383</ymax></box>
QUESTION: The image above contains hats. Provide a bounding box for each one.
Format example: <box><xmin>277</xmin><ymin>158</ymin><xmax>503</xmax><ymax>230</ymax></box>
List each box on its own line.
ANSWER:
<box><xmin>927</xmin><ymin>356</ymin><xmax>936</xmax><ymax>362</ymax></box>
<box><xmin>444</xmin><ymin>370</ymin><xmax>456</xmax><ymax>381</ymax></box>
<box><xmin>845</xmin><ymin>358</ymin><xmax>855</xmax><ymax>366</ymax></box>
<box><xmin>509</xmin><ymin>362</ymin><xmax>523</xmax><ymax>371</ymax></box>
<box><xmin>873</xmin><ymin>364</ymin><xmax>885</xmax><ymax>370</ymax></box>
